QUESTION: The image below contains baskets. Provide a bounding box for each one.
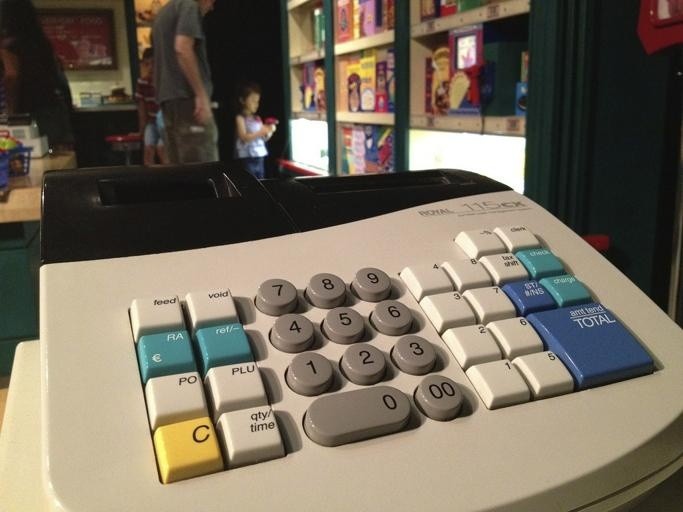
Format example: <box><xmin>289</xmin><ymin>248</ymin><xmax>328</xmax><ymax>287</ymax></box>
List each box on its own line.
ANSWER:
<box><xmin>1</xmin><ymin>130</ymin><xmax>33</xmax><ymax>187</ymax></box>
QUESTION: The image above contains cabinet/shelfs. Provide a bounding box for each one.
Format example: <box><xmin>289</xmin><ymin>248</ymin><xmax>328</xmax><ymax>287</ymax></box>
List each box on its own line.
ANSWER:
<box><xmin>409</xmin><ymin>1</ymin><xmax>589</xmax><ymax>238</ymax></box>
<box><xmin>333</xmin><ymin>0</ymin><xmax>409</xmax><ymax>180</ymax></box>
<box><xmin>278</xmin><ymin>0</ymin><xmax>335</xmax><ymax>181</ymax></box>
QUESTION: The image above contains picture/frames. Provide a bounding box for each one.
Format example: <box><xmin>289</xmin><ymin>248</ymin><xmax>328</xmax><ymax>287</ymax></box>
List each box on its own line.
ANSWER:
<box><xmin>34</xmin><ymin>9</ymin><xmax>118</xmax><ymax>73</ymax></box>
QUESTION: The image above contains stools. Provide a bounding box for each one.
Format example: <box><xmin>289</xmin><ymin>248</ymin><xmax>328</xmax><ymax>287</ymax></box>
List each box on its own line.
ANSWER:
<box><xmin>104</xmin><ymin>132</ymin><xmax>142</xmax><ymax>166</ymax></box>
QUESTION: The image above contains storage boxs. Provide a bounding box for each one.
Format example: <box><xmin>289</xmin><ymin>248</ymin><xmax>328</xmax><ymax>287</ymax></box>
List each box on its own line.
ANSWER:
<box><xmin>0</xmin><ymin>219</ymin><xmax>43</xmax><ymax>339</ymax></box>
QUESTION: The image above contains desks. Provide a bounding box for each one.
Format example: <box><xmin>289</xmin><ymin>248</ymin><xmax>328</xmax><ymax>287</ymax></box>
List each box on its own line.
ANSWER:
<box><xmin>73</xmin><ymin>102</ymin><xmax>140</xmax><ymax>139</ymax></box>
<box><xmin>0</xmin><ymin>144</ymin><xmax>78</xmax><ymax>309</ymax></box>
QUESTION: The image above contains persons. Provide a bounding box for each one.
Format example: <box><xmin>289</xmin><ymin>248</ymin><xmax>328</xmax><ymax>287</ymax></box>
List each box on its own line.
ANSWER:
<box><xmin>1</xmin><ymin>1</ymin><xmax>75</xmax><ymax>150</ymax></box>
<box><xmin>134</xmin><ymin>0</ymin><xmax>278</xmax><ymax>177</ymax></box>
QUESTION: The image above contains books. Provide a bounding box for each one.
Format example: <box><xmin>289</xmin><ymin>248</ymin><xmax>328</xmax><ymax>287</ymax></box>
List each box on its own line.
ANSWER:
<box><xmin>302</xmin><ymin>1</ymin><xmax>528</xmax><ymax>175</ymax></box>
